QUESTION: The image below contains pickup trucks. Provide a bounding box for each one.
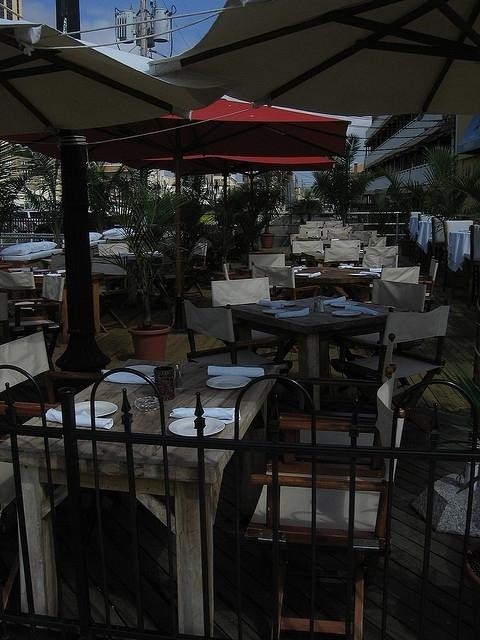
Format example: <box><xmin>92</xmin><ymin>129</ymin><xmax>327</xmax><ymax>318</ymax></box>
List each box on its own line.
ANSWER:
<box><xmin>320</xmin><ymin>203</ymin><xmax>336</xmax><ymax>216</ymax></box>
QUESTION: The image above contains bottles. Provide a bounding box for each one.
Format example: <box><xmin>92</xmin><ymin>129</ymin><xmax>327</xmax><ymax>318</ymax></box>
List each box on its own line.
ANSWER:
<box><xmin>313</xmin><ymin>298</ymin><xmax>324</xmax><ymax>314</ymax></box>
<box><xmin>174</xmin><ymin>364</ymin><xmax>185</xmax><ymax>388</ymax></box>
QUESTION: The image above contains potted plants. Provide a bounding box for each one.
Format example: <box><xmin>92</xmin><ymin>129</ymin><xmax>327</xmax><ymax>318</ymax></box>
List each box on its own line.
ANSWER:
<box><xmin>81</xmin><ymin>162</ymin><xmax>197</xmax><ymax>360</ymax></box>
<box><xmin>201</xmin><ymin>185</ymin><xmax>287</xmax><ymax>280</ymax></box>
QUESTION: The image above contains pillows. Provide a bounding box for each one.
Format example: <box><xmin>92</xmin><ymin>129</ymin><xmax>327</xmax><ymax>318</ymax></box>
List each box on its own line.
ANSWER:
<box><xmin>0</xmin><ymin>241</ymin><xmax>58</xmax><ymax>255</ymax></box>
<box><xmin>103</xmin><ymin>228</ymin><xmax>133</xmax><ymax>235</ymax></box>
<box><xmin>89</xmin><ymin>232</ymin><xmax>103</xmax><ymax>241</ymax></box>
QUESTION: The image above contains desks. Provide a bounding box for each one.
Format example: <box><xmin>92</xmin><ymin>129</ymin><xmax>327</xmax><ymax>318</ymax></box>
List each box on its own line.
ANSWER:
<box><xmin>34</xmin><ymin>272</ymin><xmax>104</xmax><ymax>344</ymax></box>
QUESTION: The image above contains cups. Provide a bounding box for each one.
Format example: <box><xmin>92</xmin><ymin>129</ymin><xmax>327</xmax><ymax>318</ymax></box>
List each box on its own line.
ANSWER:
<box><xmin>153</xmin><ymin>367</ymin><xmax>176</xmax><ymax>401</ymax></box>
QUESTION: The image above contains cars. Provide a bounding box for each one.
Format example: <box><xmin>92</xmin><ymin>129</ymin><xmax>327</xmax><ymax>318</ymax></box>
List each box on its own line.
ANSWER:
<box><xmin>3</xmin><ymin>209</ymin><xmax>54</xmax><ymax>234</ymax></box>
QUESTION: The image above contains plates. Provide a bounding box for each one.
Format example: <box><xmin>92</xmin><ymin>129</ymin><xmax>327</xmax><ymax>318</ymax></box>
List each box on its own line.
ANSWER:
<box><xmin>73</xmin><ymin>400</ymin><xmax>119</xmax><ymax>418</ymax></box>
<box><xmin>292</xmin><ymin>267</ymin><xmax>315</xmax><ymax>278</ymax></box>
<box><xmin>204</xmin><ymin>375</ymin><xmax>252</xmax><ymax>390</ymax></box>
<box><xmin>331</xmin><ymin>300</ymin><xmax>365</xmax><ymax>316</ymax></box>
<box><xmin>265</xmin><ymin>307</ymin><xmax>291</xmax><ymax>315</ymax></box>
<box><xmin>24</xmin><ymin>269</ymin><xmax>67</xmax><ymax>279</ymax></box>
<box><xmin>344</xmin><ymin>263</ymin><xmax>368</xmax><ymax>277</ymax></box>
<box><xmin>168</xmin><ymin>416</ymin><xmax>226</xmax><ymax>437</ymax></box>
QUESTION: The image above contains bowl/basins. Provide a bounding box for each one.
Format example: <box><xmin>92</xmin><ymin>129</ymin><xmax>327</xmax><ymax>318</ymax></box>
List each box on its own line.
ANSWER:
<box><xmin>133</xmin><ymin>396</ymin><xmax>161</xmax><ymax>413</ymax></box>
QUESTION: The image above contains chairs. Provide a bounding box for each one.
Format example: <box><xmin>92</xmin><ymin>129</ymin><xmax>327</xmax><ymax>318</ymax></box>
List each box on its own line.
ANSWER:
<box><xmin>0</xmin><ymin>325</ymin><xmax>104</xmax><ymax>518</ymax></box>
<box><xmin>0</xmin><ymin>272</ymin><xmax>66</xmax><ymax>325</ymax></box>
<box><xmin>97</xmin><ymin>241</ymin><xmax>130</xmax><ymax>256</ymax></box>
<box><xmin>181</xmin><ymin>221</ymin><xmax>479</xmax><ymax>422</ymax></box>
<box><xmin>249</xmin><ymin>367</ymin><xmax>405</xmax><ymax>640</ymax></box>
<box><xmin>279</xmin><ymin>333</ymin><xmax>396</xmax><ymax>446</ymax></box>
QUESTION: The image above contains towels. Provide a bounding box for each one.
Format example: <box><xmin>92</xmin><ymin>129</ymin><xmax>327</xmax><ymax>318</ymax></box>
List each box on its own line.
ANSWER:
<box><xmin>45</xmin><ymin>409</ymin><xmax>113</xmax><ymax>430</ymax></box>
<box><xmin>169</xmin><ymin>408</ymin><xmax>241</xmax><ymax>424</ymax></box>
<box><xmin>100</xmin><ymin>368</ymin><xmax>153</xmax><ymax>384</ymax></box>
<box><xmin>207</xmin><ymin>365</ymin><xmax>265</xmax><ymax>379</ymax></box>
